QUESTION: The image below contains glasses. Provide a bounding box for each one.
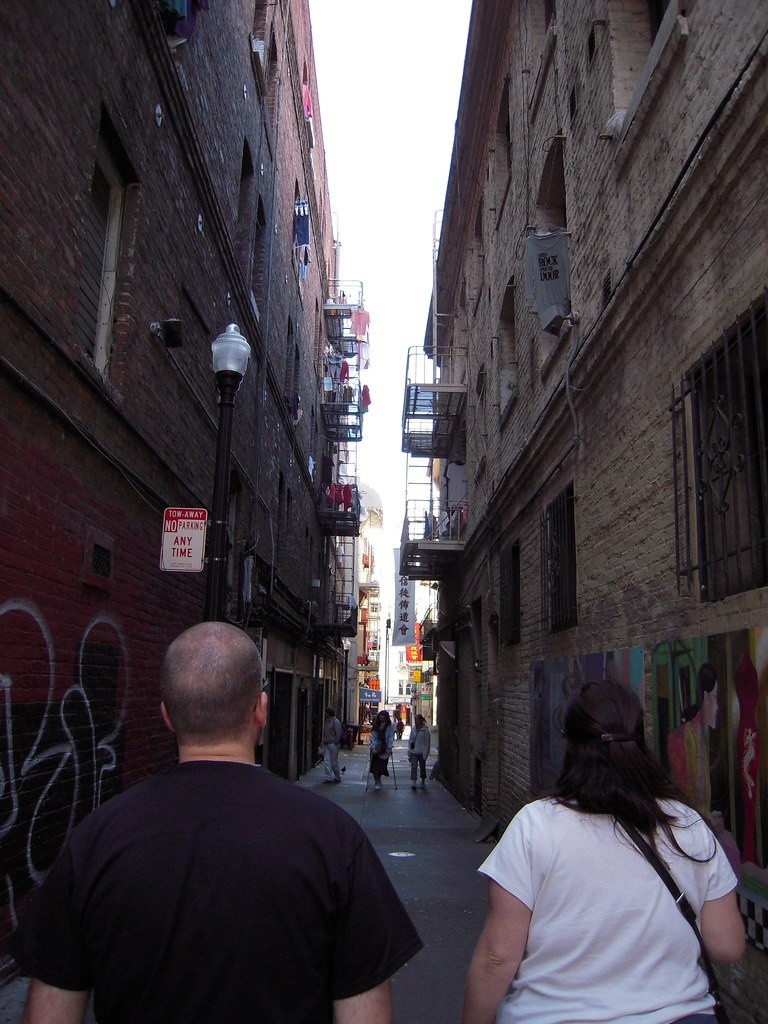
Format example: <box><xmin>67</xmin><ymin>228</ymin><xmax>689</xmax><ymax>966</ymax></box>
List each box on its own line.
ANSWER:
<box><xmin>253</xmin><ymin>678</ymin><xmax>269</xmax><ymax>712</ymax></box>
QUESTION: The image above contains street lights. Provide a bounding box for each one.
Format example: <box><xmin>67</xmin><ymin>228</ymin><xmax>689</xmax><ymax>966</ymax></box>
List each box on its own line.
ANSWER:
<box><xmin>343</xmin><ymin>639</ymin><xmax>352</xmax><ymax>746</ymax></box>
<box><xmin>203</xmin><ymin>323</ymin><xmax>251</xmax><ymax>625</ymax></box>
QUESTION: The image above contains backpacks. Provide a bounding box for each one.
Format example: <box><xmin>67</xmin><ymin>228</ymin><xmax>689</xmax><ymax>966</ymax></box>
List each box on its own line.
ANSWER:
<box><xmin>333</xmin><ymin>716</ymin><xmax>349</xmax><ymax>744</ymax></box>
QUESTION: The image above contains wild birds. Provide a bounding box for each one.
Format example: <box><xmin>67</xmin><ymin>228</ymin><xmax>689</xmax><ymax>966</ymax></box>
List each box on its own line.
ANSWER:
<box><xmin>340</xmin><ymin>766</ymin><xmax>346</xmax><ymax>773</ymax></box>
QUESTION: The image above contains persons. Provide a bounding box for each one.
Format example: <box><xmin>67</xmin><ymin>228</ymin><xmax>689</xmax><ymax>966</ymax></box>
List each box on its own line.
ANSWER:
<box><xmin>460</xmin><ymin>679</ymin><xmax>745</xmax><ymax>1024</ymax></box>
<box><xmin>407</xmin><ymin>714</ymin><xmax>430</xmax><ymax>789</ymax></box>
<box><xmin>319</xmin><ymin>706</ymin><xmax>341</xmax><ymax>784</ymax></box>
<box><xmin>391</xmin><ymin>715</ymin><xmax>405</xmax><ymax>740</ymax></box>
<box><xmin>5</xmin><ymin>619</ymin><xmax>425</xmax><ymax>1023</ymax></box>
<box><xmin>369</xmin><ymin>710</ymin><xmax>394</xmax><ymax>789</ymax></box>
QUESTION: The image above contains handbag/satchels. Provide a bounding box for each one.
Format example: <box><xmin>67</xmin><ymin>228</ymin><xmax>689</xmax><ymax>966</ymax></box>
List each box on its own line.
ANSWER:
<box><xmin>710</xmin><ymin>991</ymin><xmax>731</xmax><ymax>1024</ymax></box>
<box><xmin>376</xmin><ymin>740</ymin><xmax>387</xmax><ymax>755</ymax></box>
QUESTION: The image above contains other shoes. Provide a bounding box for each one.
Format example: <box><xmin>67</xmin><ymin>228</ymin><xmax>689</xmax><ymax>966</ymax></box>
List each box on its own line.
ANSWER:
<box><xmin>418</xmin><ymin>785</ymin><xmax>424</xmax><ymax>788</ymax></box>
<box><xmin>375</xmin><ymin>784</ymin><xmax>383</xmax><ymax>789</ymax></box>
<box><xmin>411</xmin><ymin>784</ymin><xmax>416</xmax><ymax>790</ymax></box>
<box><xmin>322</xmin><ymin>780</ymin><xmax>331</xmax><ymax>784</ymax></box>
<box><xmin>332</xmin><ymin>779</ymin><xmax>340</xmax><ymax>783</ymax></box>
